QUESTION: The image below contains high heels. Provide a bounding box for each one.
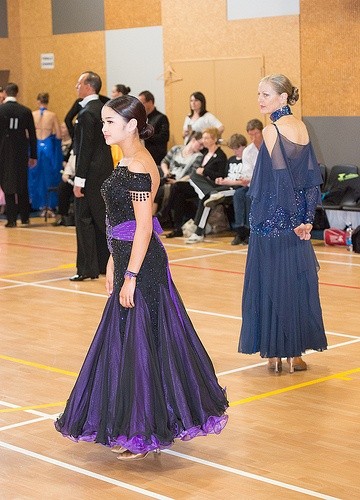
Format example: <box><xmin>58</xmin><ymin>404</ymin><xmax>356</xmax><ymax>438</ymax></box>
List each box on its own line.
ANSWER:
<box><xmin>267</xmin><ymin>355</ymin><xmax>282</xmax><ymax>372</ymax></box>
<box><xmin>287</xmin><ymin>355</ymin><xmax>307</xmax><ymax>373</ymax></box>
<box><xmin>111</xmin><ymin>444</ymin><xmax>128</xmax><ymax>453</ymax></box>
<box><xmin>115</xmin><ymin>445</ymin><xmax>162</xmax><ymax>460</ymax></box>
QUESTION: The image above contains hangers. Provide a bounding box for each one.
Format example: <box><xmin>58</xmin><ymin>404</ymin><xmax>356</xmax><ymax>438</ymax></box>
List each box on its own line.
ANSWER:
<box><xmin>156</xmin><ymin>61</ymin><xmax>184</xmax><ymax>81</ymax></box>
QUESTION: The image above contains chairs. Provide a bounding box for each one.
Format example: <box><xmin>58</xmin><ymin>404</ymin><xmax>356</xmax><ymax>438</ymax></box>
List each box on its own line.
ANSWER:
<box><xmin>317</xmin><ymin>163</ymin><xmax>360</xmax><ymax>210</ymax></box>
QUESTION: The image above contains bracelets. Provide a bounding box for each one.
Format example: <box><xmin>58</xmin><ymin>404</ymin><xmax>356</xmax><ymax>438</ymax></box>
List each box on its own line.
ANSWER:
<box><xmin>123</xmin><ymin>270</ymin><xmax>138</xmax><ymax>279</ymax></box>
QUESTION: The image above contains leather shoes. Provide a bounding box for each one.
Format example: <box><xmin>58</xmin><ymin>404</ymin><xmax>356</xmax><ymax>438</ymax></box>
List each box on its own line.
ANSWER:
<box><xmin>70</xmin><ymin>274</ymin><xmax>99</xmax><ymax>281</ymax></box>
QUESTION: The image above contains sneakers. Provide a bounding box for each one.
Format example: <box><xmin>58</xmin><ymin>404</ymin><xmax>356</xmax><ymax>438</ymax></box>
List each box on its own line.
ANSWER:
<box><xmin>185</xmin><ymin>232</ymin><xmax>204</xmax><ymax>243</ymax></box>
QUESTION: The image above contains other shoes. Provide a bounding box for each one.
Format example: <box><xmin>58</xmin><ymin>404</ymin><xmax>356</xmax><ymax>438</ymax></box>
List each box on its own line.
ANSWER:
<box><xmin>167</xmin><ymin>230</ymin><xmax>184</xmax><ymax>237</ymax></box>
<box><xmin>152</xmin><ymin>179</ymin><xmax>163</xmax><ymax>222</ymax></box>
<box><xmin>55</xmin><ymin>217</ymin><xmax>65</xmax><ymax>225</ymax></box>
<box><xmin>5</xmin><ymin>221</ymin><xmax>16</xmax><ymax>227</ymax></box>
<box><xmin>47</xmin><ymin>210</ymin><xmax>55</xmax><ymax>217</ymax></box>
<box><xmin>22</xmin><ymin>216</ymin><xmax>30</xmax><ymax>224</ymax></box>
<box><xmin>41</xmin><ymin>210</ymin><xmax>46</xmax><ymax>217</ymax></box>
<box><xmin>232</xmin><ymin>231</ymin><xmax>246</xmax><ymax>244</ymax></box>
<box><xmin>241</xmin><ymin>236</ymin><xmax>250</xmax><ymax>245</ymax></box>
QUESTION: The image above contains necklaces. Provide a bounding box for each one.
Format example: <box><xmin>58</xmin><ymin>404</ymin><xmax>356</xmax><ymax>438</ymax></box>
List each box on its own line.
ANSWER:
<box><xmin>39</xmin><ymin>107</ymin><xmax>49</xmax><ymax>116</ymax></box>
<box><xmin>269</xmin><ymin>105</ymin><xmax>294</xmax><ymax>122</ymax></box>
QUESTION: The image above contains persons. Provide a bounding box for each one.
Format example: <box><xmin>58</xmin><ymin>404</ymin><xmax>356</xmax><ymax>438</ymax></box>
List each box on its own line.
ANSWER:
<box><xmin>27</xmin><ymin>92</ymin><xmax>64</xmax><ymax>218</ymax></box>
<box><xmin>230</xmin><ymin>119</ymin><xmax>264</xmax><ymax>245</ymax></box>
<box><xmin>153</xmin><ymin>130</ymin><xmax>203</xmax><ymax>216</ymax></box>
<box><xmin>55</xmin><ymin>93</ymin><xmax>229</xmax><ymax>462</ymax></box>
<box><xmin>182</xmin><ymin>92</ymin><xmax>225</xmax><ymax>146</ymax></box>
<box><xmin>112</xmin><ymin>84</ymin><xmax>131</xmax><ymax>101</ymax></box>
<box><xmin>185</xmin><ymin>133</ymin><xmax>248</xmax><ymax>245</ymax></box>
<box><xmin>69</xmin><ymin>71</ymin><xmax>114</xmax><ymax>282</ymax></box>
<box><xmin>238</xmin><ymin>74</ymin><xmax>328</xmax><ymax>373</ymax></box>
<box><xmin>137</xmin><ymin>91</ymin><xmax>169</xmax><ymax>166</ymax></box>
<box><xmin>59</xmin><ymin>121</ymin><xmax>72</xmax><ymax>157</ymax></box>
<box><xmin>51</xmin><ymin>94</ymin><xmax>111</xmax><ymax>227</ymax></box>
<box><xmin>152</xmin><ymin>126</ymin><xmax>227</xmax><ymax>238</ymax></box>
<box><xmin>0</xmin><ymin>87</ymin><xmax>19</xmax><ymax>216</ymax></box>
<box><xmin>0</xmin><ymin>83</ymin><xmax>38</xmax><ymax>227</ymax></box>
